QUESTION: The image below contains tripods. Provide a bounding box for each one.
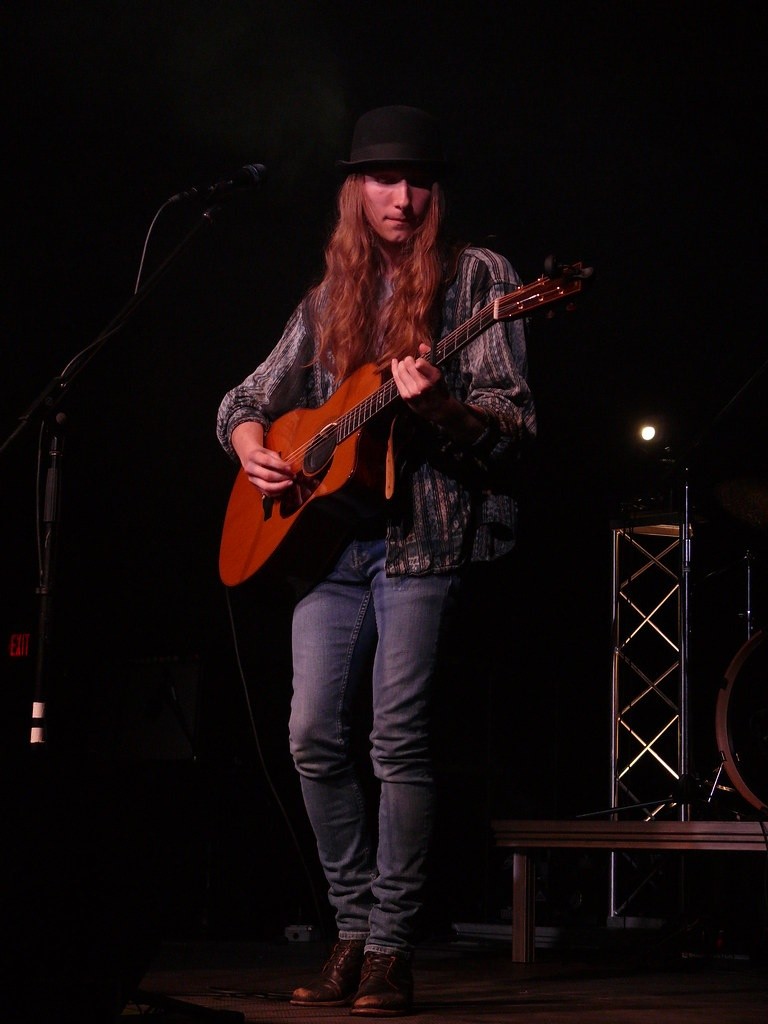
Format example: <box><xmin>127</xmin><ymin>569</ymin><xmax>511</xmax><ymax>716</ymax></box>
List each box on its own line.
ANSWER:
<box><xmin>575</xmin><ymin>361</ymin><xmax>768</xmax><ymax>823</ymax></box>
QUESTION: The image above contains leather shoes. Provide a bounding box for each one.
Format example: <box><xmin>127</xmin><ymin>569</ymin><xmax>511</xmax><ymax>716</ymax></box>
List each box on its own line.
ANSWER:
<box><xmin>289</xmin><ymin>941</ymin><xmax>365</xmax><ymax>1007</ymax></box>
<box><xmin>349</xmin><ymin>952</ymin><xmax>414</xmax><ymax>1018</ymax></box>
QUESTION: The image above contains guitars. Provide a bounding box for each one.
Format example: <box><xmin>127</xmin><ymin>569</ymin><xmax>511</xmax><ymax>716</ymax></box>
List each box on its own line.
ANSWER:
<box><xmin>217</xmin><ymin>263</ymin><xmax>594</xmax><ymax>599</ymax></box>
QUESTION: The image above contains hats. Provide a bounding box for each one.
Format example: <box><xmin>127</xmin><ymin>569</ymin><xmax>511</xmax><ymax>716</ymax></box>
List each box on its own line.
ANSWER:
<box><xmin>334</xmin><ymin>104</ymin><xmax>462</xmax><ymax>175</ymax></box>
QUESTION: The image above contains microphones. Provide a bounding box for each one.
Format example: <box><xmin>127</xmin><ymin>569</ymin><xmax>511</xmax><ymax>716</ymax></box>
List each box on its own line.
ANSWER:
<box><xmin>170</xmin><ymin>164</ymin><xmax>268</xmax><ymax>204</ymax></box>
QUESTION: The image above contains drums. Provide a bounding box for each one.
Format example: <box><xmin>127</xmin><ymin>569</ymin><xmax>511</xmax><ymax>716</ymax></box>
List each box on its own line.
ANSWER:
<box><xmin>716</xmin><ymin>626</ymin><xmax>767</xmax><ymax>819</ymax></box>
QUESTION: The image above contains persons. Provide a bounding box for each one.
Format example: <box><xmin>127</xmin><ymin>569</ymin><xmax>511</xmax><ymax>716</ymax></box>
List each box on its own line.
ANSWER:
<box><xmin>216</xmin><ymin>105</ymin><xmax>540</xmax><ymax>1014</ymax></box>
